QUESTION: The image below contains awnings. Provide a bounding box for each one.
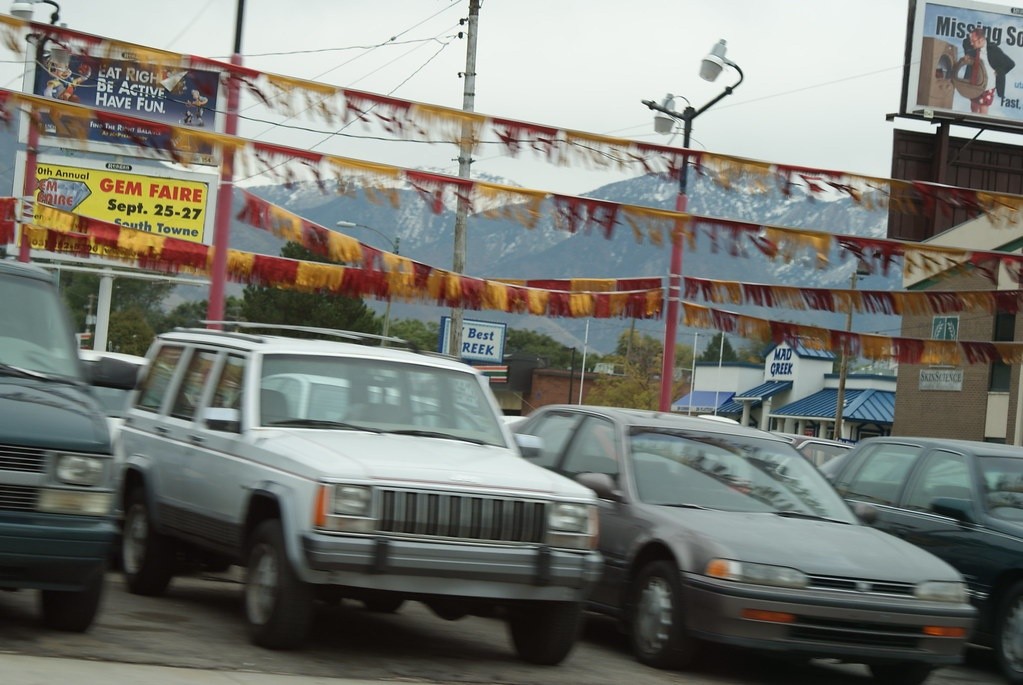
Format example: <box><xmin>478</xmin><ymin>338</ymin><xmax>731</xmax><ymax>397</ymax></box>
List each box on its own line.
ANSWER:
<box><xmin>672</xmin><ymin>391</ymin><xmax>761</xmax><ymax>416</ymax></box>
<box><xmin>472</xmin><ymin>359</ymin><xmax>530</xmax><ymax>392</ymax></box>
<box><xmin>733</xmin><ymin>381</ymin><xmax>792</xmax><ymax>432</ymax></box>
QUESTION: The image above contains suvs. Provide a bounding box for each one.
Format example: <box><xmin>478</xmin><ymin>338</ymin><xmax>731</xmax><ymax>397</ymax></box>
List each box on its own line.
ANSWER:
<box><xmin>0</xmin><ymin>254</ymin><xmax>140</xmax><ymax>637</ymax></box>
<box><xmin>110</xmin><ymin>319</ymin><xmax>605</xmax><ymax>665</ymax></box>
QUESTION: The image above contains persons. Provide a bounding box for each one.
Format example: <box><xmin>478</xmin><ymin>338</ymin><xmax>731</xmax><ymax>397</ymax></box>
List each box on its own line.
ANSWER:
<box><xmin>961</xmin><ymin>29</ymin><xmax>1015</xmax><ymax>114</ymax></box>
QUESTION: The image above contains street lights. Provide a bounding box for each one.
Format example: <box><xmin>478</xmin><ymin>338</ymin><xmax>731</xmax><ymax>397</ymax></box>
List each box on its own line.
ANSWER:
<box><xmin>637</xmin><ymin>37</ymin><xmax>745</xmax><ymax>418</ymax></box>
<box><xmin>560</xmin><ymin>343</ymin><xmax>577</xmax><ymax>404</ymax></box>
<box><xmin>1</xmin><ymin>0</ymin><xmax>63</xmax><ymax>264</ymax></box>
<box><xmin>335</xmin><ymin>217</ymin><xmax>402</xmax><ymax>347</ymax></box>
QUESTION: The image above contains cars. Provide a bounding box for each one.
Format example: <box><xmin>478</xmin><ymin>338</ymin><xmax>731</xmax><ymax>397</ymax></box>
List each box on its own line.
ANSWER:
<box><xmin>507</xmin><ymin>402</ymin><xmax>977</xmax><ymax>685</ymax></box>
<box><xmin>830</xmin><ymin>433</ymin><xmax>1023</xmax><ymax>685</ymax></box>
<box><xmin>226</xmin><ymin>371</ymin><xmax>488</xmax><ymax>435</ymax></box>
<box><xmin>79</xmin><ymin>343</ymin><xmax>199</xmax><ymax>442</ymax></box>
<box><xmin>497</xmin><ymin>411</ymin><xmax>914</xmax><ymax>490</ymax></box>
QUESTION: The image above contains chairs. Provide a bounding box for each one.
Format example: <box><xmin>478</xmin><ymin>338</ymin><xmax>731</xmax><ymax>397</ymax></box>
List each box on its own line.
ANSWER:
<box><xmin>343</xmin><ymin>402</ymin><xmax>413</xmax><ymax>422</ymax></box>
<box><xmin>230</xmin><ymin>389</ymin><xmax>289</xmax><ymax>421</ymax></box>
